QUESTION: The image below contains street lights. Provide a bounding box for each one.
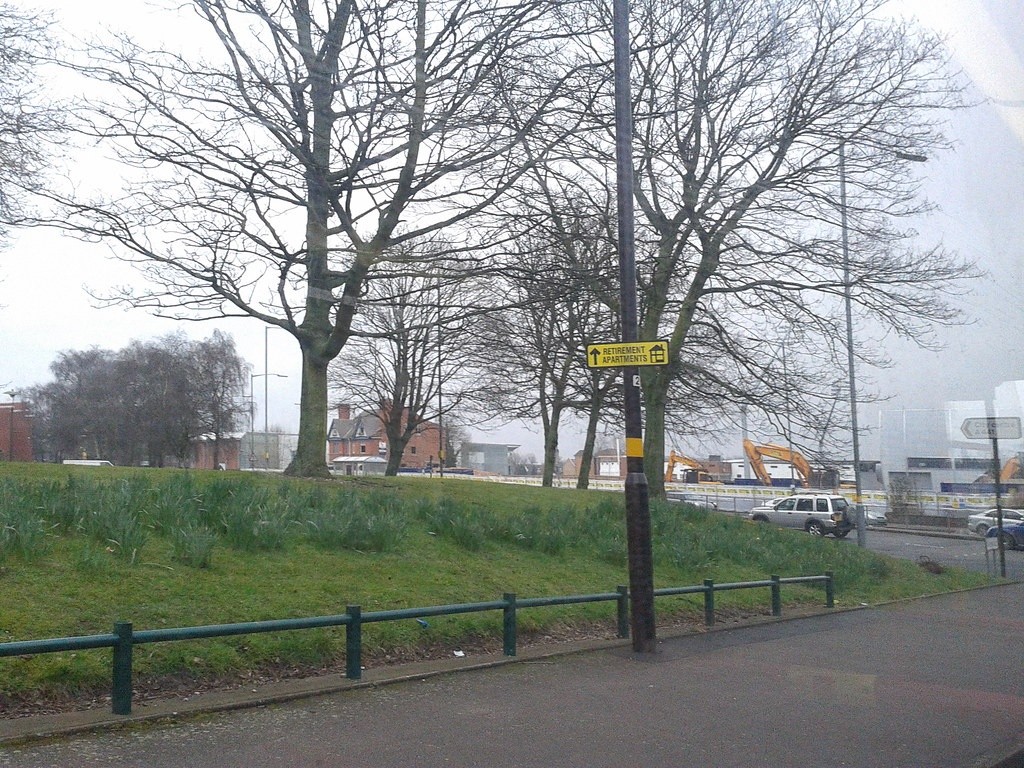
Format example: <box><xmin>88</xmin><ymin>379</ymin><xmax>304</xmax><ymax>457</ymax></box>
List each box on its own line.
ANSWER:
<box><xmin>251</xmin><ymin>373</ymin><xmax>289</xmax><ymax>470</ymax></box>
<box><xmin>4</xmin><ymin>388</ymin><xmax>20</xmax><ymax>463</ymax></box>
<box><xmin>836</xmin><ymin>135</ymin><xmax>928</xmax><ymax>550</ymax></box>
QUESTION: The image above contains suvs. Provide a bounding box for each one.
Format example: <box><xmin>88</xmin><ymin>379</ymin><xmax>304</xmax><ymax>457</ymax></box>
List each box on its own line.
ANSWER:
<box><xmin>748</xmin><ymin>491</ymin><xmax>857</xmax><ymax>537</ymax></box>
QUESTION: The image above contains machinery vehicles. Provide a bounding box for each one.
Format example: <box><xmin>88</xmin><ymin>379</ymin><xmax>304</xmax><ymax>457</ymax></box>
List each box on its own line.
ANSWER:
<box><xmin>743</xmin><ymin>437</ymin><xmax>857</xmax><ymax>490</ymax></box>
<box><xmin>663</xmin><ymin>449</ymin><xmax>726</xmax><ymax>485</ymax></box>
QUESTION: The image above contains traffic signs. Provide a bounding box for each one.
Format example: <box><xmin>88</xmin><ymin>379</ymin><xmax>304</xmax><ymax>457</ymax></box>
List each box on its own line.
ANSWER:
<box><xmin>586</xmin><ymin>338</ymin><xmax>670</xmax><ymax>368</ymax></box>
<box><xmin>961</xmin><ymin>416</ymin><xmax>1022</xmax><ymax>440</ymax></box>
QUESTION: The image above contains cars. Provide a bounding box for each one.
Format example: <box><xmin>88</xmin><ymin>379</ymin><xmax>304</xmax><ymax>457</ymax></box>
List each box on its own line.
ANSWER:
<box><xmin>864</xmin><ymin>507</ymin><xmax>889</xmax><ymax>527</ymax></box>
<box><xmin>969</xmin><ymin>507</ymin><xmax>1024</xmax><ymax>536</ymax></box>
<box><xmin>666</xmin><ymin>489</ymin><xmax>717</xmax><ymax>512</ymax></box>
<box><xmin>761</xmin><ymin>497</ymin><xmax>797</xmax><ymax>509</ymax></box>
<box><xmin>985</xmin><ymin>521</ymin><xmax>1024</xmax><ymax>550</ymax></box>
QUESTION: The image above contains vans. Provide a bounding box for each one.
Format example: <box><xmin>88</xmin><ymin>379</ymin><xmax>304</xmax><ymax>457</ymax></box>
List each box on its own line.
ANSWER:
<box><xmin>63</xmin><ymin>459</ymin><xmax>114</xmax><ymax>466</ymax></box>
